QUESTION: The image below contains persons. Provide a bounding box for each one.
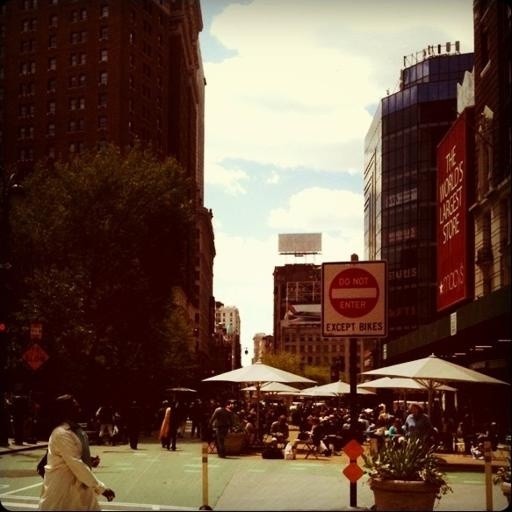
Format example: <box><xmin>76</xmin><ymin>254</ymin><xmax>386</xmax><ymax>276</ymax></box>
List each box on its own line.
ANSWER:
<box><xmin>36</xmin><ymin>394</ymin><xmax>117</xmax><ymax>511</ymax></box>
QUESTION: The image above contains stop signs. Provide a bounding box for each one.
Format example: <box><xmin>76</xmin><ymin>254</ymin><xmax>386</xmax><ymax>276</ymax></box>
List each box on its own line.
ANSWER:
<box><xmin>321</xmin><ymin>261</ymin><xmax>387</xmax><ymax>337</ymax></box>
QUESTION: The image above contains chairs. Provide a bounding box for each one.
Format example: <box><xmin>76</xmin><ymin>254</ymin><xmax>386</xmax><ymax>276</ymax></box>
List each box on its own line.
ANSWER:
<box><xmin>305</xmin><ymin>433</ymin><xmax>325</xmax><ymax>459</ymax></box>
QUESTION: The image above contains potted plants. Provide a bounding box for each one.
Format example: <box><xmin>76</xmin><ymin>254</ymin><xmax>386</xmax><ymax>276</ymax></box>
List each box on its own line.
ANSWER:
<box><xmin>363</xmin><ymin>433</ymin><xmax>453</xmax><ymax>512</ymax></box>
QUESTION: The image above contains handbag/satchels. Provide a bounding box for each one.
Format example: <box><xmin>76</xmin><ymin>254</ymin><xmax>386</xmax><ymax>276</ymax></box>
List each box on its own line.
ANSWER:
<box><xmin>37</xmin><ymin>452</ymin><xmax>47</xmax><ymax>478</ymax></box>
